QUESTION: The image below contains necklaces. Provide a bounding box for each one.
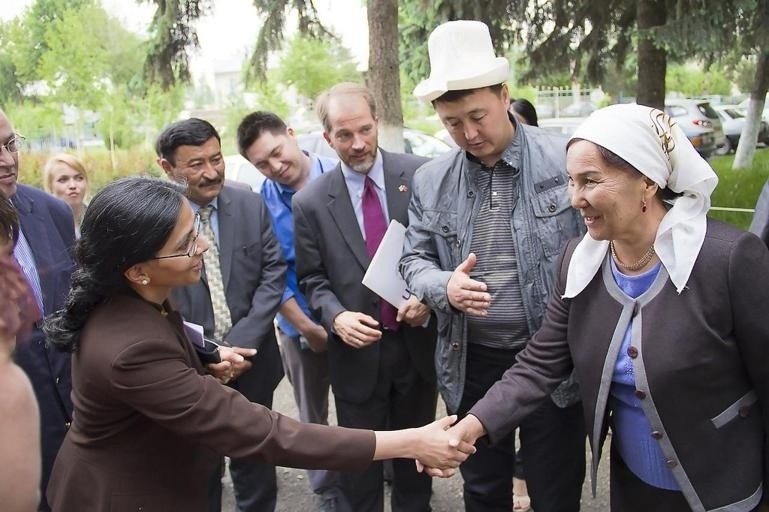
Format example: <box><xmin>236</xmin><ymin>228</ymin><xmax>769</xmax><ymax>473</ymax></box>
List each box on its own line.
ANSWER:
<box><xmin>608</xmin><ymin>240</ymin><xmax>654</xmax><ymax>271</ymax></box>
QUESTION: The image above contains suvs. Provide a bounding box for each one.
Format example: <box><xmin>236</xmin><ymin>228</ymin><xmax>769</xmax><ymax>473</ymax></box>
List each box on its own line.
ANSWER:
<box><xmin>661</xmin><ymin>99</ymin><xmax>725</xmax><ymax>152</ymax></box>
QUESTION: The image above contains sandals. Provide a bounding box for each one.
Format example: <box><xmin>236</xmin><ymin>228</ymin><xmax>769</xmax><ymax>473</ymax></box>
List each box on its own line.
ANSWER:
<box><xmin>513</xmin><ymin>494</ymin><xmax>531</xmax><ymax>511</ymax></box>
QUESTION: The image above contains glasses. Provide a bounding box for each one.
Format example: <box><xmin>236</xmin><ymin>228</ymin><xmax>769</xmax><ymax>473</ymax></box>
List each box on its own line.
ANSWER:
<box><xmin>1</xmin><ymin>133</ymin><xmax>25</xmax><ymax>153</ymax></box>
<box><xmin>140</xmin><ymin>211</ymin><xmax>201</xmax><ymax>264</ymax></box>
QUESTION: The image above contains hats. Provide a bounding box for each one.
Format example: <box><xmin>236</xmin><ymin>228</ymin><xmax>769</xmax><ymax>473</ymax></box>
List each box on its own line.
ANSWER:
<box><xmin>414</xmin><ymin>21</ymin><xmax>509</xmax><ymax>100</ymax></box>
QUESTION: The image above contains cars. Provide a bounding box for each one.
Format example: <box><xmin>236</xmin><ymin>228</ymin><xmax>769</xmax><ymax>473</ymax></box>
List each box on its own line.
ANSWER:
<box><xmin>560</xmin><ymin>101</ymin><xmax>597</xmax><ymax>118</ymax></box>
<box><xmin>710</xmin><ymin>104</ymin><xmax>768</xmax><ymax>155</ymax></box>
<box><xmin>225</xmin><ymin>124</ymin><xmax>453</xmax><ymax>191</ymax></box>
<box><xmin>525</xmin><ymin>118</ymin><xmax>586</xmax><ymax>152</ymax></box>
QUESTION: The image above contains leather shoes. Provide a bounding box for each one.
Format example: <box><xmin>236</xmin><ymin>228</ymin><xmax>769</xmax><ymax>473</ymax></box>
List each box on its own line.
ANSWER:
<box><xmin>317</xmin><ymin>494</ymin><xmax>339</xmax><ymax>511</ymax></box>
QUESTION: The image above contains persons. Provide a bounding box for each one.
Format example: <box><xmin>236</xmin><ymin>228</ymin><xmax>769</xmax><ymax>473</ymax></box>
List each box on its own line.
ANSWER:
<box><xmin>509</xmin><ymin>98</ymin><xmax>538</xmax><ymax>127</ymax></box>
<box><xmin>293</xmin><ymin>83</ymin><xmax>438</xmax><ymax>512</ymax></box>
<box><xmin>0</xmin><ymin>186</ymin><xmax>41</xmax><ymax>511</ymax></box>
<box><xmin>0</xmin><ymin>109</ymin><xmax>78</xmax><ymax>512</ymax></box>
<box><xmin>44</xmin><ymin>180</ymin><xmax>478</xmax><ymax>512</ymax></box>
<box><xmin>153</xmin><ymin>116</ymin><xmax>290</xmax><ymax>512</ymax></box>
<box><xmin>238</xmin><ymin>113</ymin><xmax>342</xmax><ymax>511</ymax></box>
<box><xmin>510</xmin><ymin>446</ymin><xmax>532</xmax><ymax>511</ymax></box>
<box><xmin>400</xmin><ymin>83</ymin><xmax>587</xmax><ymax>512</ymax></box>
<box><xmin>43</xmin><ymin>155</ymin><xmax>90</xmax><ymax>242</ymax></box>
<box><xmin>415</xmin><ymin>103</ymin><xmax>768</xmax><ymax>512</ymax></box>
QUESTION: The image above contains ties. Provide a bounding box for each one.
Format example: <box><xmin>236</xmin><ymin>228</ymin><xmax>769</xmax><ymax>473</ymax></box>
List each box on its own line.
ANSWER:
<box><xmin>198</xmin><ymin>206</ymin><xmax>232</xmax><ymax>345</ymax></box>
<box><xmin>362</xmin><ymin>176</ymin><xmax>401</xmax><ymax>334</ymax></box>
